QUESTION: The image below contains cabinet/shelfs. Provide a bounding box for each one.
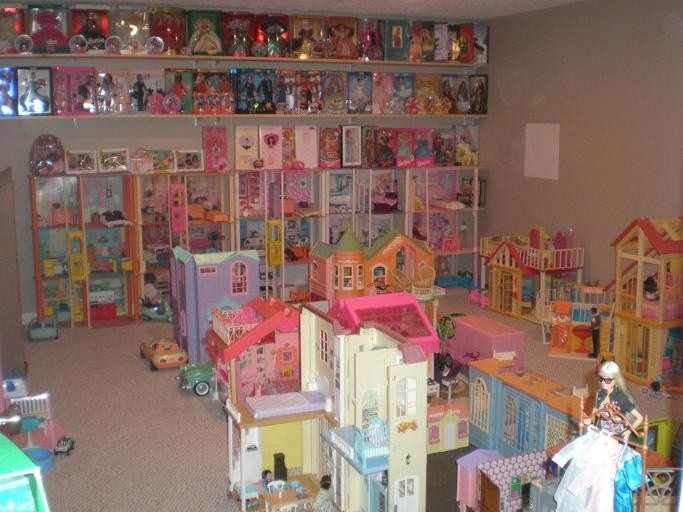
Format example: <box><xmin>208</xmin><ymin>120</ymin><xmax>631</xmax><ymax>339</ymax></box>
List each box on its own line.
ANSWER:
<box><xmin>1</xmin><ymin>54</ymin><xmax>490</xmax><ymax>121</ymax></box>
